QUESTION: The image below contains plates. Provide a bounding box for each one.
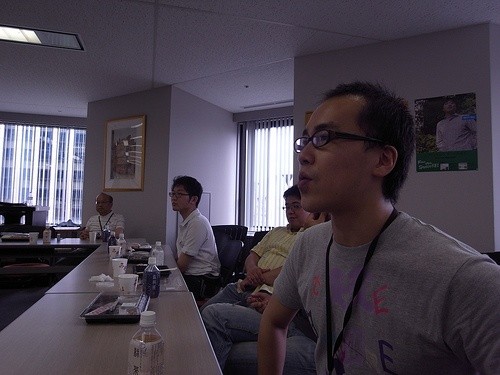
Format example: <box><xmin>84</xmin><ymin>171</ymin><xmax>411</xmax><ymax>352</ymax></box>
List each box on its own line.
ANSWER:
<box><xmin>121</xmin><ymin>247</ymin><xmax>172</xmax><ymax>279</ymax></box>
<box><xmin>79</xmin><ymin>294</ymin><xmax>151</xmax><ymax>323</ymax></box>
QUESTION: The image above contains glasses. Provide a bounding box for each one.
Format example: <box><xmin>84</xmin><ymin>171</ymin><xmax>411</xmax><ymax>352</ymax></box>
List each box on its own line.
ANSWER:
<box><xmin>294</xmin><ymin>129</ymin><xmax>387</xmax><ymax>153</ymax></box>
<box><xmin>283</xmin><ymin>203</ymin><xmax>302</xmax><ymax>211</ymax></box>
<box><xmin>168</xmin><ymin>191</ymin><xmax>191</xmax><ymax>198</ymax></box>
<box><xmin>95</xmin><ymin>201</ymin><xmax>109</xmax><ymax>205</ymax></box>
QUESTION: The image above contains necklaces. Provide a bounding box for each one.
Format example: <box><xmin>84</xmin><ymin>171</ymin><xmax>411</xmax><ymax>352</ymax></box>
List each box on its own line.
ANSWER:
<box><xmin>325</xmin><ymin>207</ymin><xmax>398</xmax><ymax>375</ymax></box>
<box><xmin>99</xmin><ymin>213</ymin><xmax>114</xmax><ymax>230</ymax></box>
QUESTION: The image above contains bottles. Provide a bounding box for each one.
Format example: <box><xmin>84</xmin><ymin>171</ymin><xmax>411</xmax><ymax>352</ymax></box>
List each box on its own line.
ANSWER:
<box><xmin>152</xmin><ymin>240</ymin><xmax>164</xmax><ymax>265</ymax></box>
<box><xmin>128</xmin><ymin>311</ymin><xmax>164</xmax><ymax>375</ymax></box>
<box><xmin>102</xmin><ymin>224</ymin><xmax>126</xmax><ymax>255</ymax></box>
<box><xmin>143</xmin><ymin>257</ymin><xmax>161</xmax><ymax>298</ymax></box>
<box><xmin>43</xmin><ymin>226</ymin><xmax>52</xmax><ymax>243</ymax></box>
<box><xmin>27</xmin><ymin>192</ymin><xmax>34</xmax><ymax>207</ymax></box>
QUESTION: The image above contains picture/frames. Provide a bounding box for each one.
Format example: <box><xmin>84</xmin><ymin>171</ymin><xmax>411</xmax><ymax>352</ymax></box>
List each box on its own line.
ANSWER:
<box><xmin>103</xmin><ymin>113</ymin><xmax>146</xmax><ymax>192</ymax></box>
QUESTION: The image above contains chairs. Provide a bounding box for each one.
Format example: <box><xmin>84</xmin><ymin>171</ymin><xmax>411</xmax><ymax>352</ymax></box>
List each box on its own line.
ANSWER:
<box><xmin>196</xmin><ymin>233</ymin><xmax>245</xmax><ymax>300</ymax></box>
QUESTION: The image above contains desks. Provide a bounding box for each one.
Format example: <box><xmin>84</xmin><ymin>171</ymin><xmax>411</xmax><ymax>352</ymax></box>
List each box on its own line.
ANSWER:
<box><xmin>0</xmin><ymin>290</ymin><xmax>223</xmax><ymax>375</ymax></box>
<box><xmin>0</xmin><ymin>234</ymin><xmax>147</xmax><ymax>266</ymax></box>
<box><xmin>45</xmin><ymin>241</ymin><xmax>189</xmax><ymax>294</ymax></box>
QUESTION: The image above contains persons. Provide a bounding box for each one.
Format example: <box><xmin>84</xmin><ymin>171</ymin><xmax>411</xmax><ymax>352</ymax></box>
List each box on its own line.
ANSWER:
<box><xmin>258</xmin><ymin>81</ymin><xmax>500</xmax><ymax>375</ymax></box>
<box><xmin>436</xmin><ymin>95</ymin><xmax>477</xmax><ymax>151</ymax></box>
<box><xmin>200</xmin><ymin>184</ymin><xmax>311</xmax><ymax>309</ymax></box>
<box><xmin>57</xmin><ymin>192</ymin><xmax>126</xmax><ymax>256</ymax></box>
<box><xmin>201</xmin><ymin>212</ymin><xmax>328</xmax><ymax>375</ymax></box>
<box><xmin>168</xmin><ymin>176</ymin><xmax>221</xmax><ymax>301</ymax></box>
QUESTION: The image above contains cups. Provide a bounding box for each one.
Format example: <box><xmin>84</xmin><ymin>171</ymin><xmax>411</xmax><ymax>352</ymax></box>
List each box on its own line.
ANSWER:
<box><xmin>29</xmin><ymin>232</ymin><xmax>39</xmax><ymax>243</ymax></box>
<box><xmin>108</xmin><ymin>245</ymin><xmax>139</xmax><ymax>293</ymax></box>
<box><xmin>89</xmin><ymin>232</ymin><xmax>97</xmax><ymax>242</ymax></box>
